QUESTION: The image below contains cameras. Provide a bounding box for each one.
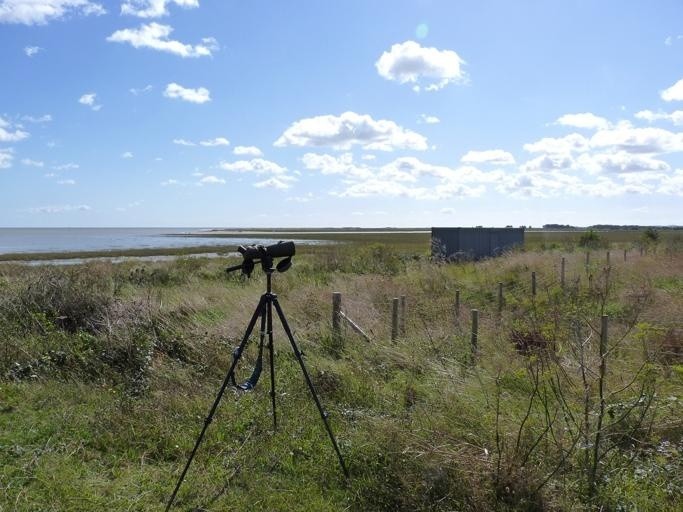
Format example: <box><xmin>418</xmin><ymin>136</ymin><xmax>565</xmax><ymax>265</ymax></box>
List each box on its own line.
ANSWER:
<box><xmin>224</xmin><ymin>239</ymin><xmax>295</xmax><ymax>279</ymax></box>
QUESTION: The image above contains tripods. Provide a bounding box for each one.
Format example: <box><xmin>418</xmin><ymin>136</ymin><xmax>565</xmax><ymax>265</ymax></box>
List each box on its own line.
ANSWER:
<box><xmin>165</xmin><ymin>258</ymin><xmax>351</xmax><ymax>512</ymax></box>
